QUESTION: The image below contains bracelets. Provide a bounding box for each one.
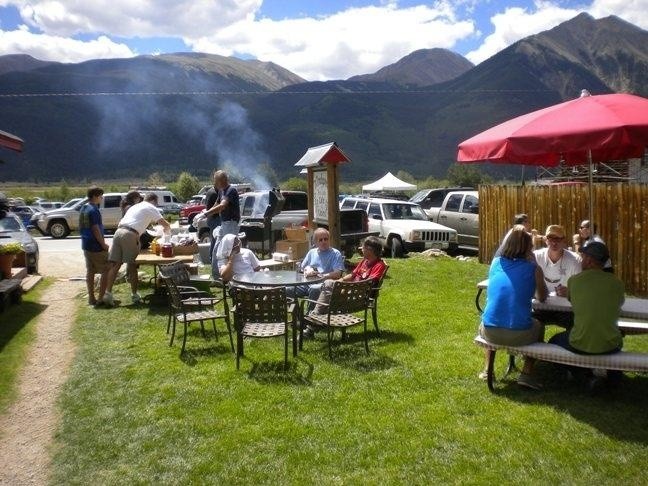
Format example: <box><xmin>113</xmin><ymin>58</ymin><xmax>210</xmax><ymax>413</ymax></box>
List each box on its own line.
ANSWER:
<box><xmin>574</xmin><ymin>240</ymin><xmax>582</xmax><ymax>246</ymax></box>
<box><xmin>341</xmin><ymin>276</ymin><xmax>345</xmax><ymax>282</ymax></box>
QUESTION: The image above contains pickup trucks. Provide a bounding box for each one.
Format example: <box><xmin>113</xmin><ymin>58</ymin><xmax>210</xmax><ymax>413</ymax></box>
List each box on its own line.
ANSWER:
<box><xmin>192</xmin><ymin>191</ymin><xmax>379</xmax><ymax>252</ymax></box>
<box><xmin>432</xmin><ymin>191</ymin><xmax>480</xmax><ymax>248</ymax></box>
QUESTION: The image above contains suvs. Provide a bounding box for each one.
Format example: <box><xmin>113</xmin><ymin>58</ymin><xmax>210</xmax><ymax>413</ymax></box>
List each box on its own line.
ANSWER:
<box><xmin>406</xmin><ymin>187</ymin><xmax>475</xmax><ymax>209</ymax></box>
<box><xmin>38</xmin><ymin>193</ymin><xmax>146</xmax><ymax>239</ymax></box>
<box><xmin>339</xmin><ymin>197</ymin><xmax>459</xmax><ymax>258</ymax></box>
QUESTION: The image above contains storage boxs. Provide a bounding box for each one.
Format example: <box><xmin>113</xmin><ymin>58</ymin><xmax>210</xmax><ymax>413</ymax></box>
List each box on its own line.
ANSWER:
<box><xmin>155</xmin><ymin>263</ymin><xmax>212</xmax><ymax>296</ymax></box>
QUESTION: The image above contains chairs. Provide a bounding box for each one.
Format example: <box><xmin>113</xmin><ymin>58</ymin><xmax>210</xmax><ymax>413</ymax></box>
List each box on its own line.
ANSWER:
<box><xmin>159</xmin><ymin>259</ymin><xmax>217</xmax><ymax>336</ymax></box>
<box><xmin>229</xmin><ymin>287</ymin><xmax>297</xmax><ymax>369</ymax></box>
<box><xmin>161</xmin><ymin>275</ymin><xmax>235</xmax><ymax>355</ymax></box>
<box><xmin>340</xmin><ymin>258</ymin><xmax>389</xmax><ymax>338</ymax></box>
<box><xmin>299</xmin><ymin>279</ymin><xmax>373</xmax><ymax>359</ymax></box>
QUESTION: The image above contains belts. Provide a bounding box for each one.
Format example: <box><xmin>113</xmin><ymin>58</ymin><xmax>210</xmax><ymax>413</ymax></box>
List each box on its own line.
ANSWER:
<box><xmin>119</xmin><ymin>225</ymin><xmax>139</xmax><ymax>236</ymax></box>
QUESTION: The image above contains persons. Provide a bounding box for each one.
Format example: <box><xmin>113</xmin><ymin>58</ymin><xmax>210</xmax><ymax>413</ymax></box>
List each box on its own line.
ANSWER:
<box><xmin>215</xmin><ymin>233</ymin><xmax>262</xmax><ymax>308</ymax></box>
<box><xmin>548</xmin><ymin>240</ymin><xmax>626</xmax><ymax>391</ymax></box>
<box><xmin>289</xmin><ymin>236</ymin><xmax>385</xmax><ymax>342</ymax></box>
<box><xmin>572</xmin><ymin>220</ymin><xmax>614</xmax><ymax>273</ymax></box>
<box><xmin>283</xmin><ymin>228</ymin><xmax>344</xmax><ymax>331</ymax></box>
<box><xmin>479</xmin><ymin>228</ymin><xmax>548</xmax><ymax>392</ymax></box>
<box><xmin>491</xmin><ymin>214</ymin><xmax>544</xmax><ymax>261</ymax></box>
<box><xmin>75</xmin><ymin>170</ymin><xmax>241</xmax><ymax>307</ymax></box>
<box><xmin>533</xmin><ymin>223</ymin><xmax>583</xmax><ymax>331</ymax></box>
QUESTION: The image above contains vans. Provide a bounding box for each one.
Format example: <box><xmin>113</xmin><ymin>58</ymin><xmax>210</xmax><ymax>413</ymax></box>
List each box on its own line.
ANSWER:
<box><xmin>127</xmin><ymin>189</ymin><xmax>186</xmax><ymax>216</ymax></box>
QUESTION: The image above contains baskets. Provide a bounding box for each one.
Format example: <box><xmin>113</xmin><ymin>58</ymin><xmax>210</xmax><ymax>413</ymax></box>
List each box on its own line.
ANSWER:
<box><xmin>174</xmin><ymin>243</ymin><xmax>199</xmax><ymax>255</ymax></box>
<box><xmin>284</xmin><ymin>227</ymin><xmax>306</xmax><ymax>241</ymax></box>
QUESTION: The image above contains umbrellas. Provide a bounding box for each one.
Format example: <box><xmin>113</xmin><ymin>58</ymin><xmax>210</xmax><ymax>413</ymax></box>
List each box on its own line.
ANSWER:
<box><xmin>456</xmin><ymin>90</ymin><xmax>648</xmax><ymax>241</ymax></box>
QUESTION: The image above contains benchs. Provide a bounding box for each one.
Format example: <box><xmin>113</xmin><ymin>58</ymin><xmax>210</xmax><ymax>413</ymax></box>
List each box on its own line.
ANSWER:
<box><xmin>474</xmin><ymin>334</ymin><xmax>647</xmax><ymax>388</ymax></box>
<box><xmin>476</xmin><ymin>279</ymin><xmax>488</xmax><ymax>314</ymax></box>
<box><xmin>617</xmin><ymin>320</ymin><xmax>648</xmax><ymax>334</ymax></box>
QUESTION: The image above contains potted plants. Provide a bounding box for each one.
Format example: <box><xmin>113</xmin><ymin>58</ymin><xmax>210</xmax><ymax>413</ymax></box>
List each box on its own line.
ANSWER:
<box><xmin>1</xmin><ymin>243</ymin><xmax>26</xmax><ymax>278</ymax></box>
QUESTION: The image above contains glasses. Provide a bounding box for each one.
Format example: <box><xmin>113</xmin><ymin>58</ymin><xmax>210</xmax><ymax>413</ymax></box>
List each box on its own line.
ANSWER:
<box><xmin>547</xmin><ymin>237</ymin><xmax>565</xmax><ymax>243</ymax></box>
<box><xmin>580</xmin><ymin>227</ymin><xmax>588</xmax><ymax>229</ymax></box>
<box><xmin>317</xmin><ymin>237</ymin><xmax>329</xmax><ymax>242</ymax></box>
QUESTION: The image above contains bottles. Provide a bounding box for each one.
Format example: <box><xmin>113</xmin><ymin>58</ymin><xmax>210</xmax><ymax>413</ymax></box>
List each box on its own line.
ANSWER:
<box><xmin>151</xmin><ymin>241</ymin><xmax>160</xmax><ymax>255</ymax></box>
<box><xmin>288</xmin><ymin>247</ymin><xmax>294</xmax><ymax>261</ymax></box>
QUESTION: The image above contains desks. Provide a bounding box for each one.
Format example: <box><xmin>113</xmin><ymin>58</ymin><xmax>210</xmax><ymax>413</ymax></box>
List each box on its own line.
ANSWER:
<box><xmin>256</xmin><ymin>258</ymin><xmax>300</xmax><ymax>271</ymax></box>
<box><xmin>135</xmin><ymin>249</ymin><xmax>194</xmax><ymax>303</ymax></box>
<box><xmin>233</xmin><ymin>268</ymin><xmax>322</xmax><ymax>284</ymax></box>
<box><xmin>532</xmin><ymin>288</ymin><xmax>648</xmax><ymax>321</ymax></box>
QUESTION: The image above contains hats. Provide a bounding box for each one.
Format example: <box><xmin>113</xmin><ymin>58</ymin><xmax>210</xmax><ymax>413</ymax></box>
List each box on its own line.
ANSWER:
<box><xmin>578</xmin><ymin>242</ymin><xmax>610</xmax><ymax>262</ymax></box>
<box><xmin>545</xmin><ymin>225</ymin><xmax>566</xmax><ymax>238</ymax></box>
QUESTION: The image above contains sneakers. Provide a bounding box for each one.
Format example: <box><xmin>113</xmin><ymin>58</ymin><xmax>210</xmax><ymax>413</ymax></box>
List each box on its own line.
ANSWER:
<box><xmin>303</xmin><ymin>328</ymin><xmax>314</xmax><ymax>339</ymax></box>
<box><xmin>102</xmin><ymin>292</ymin><xmax>115</xmax><ymax>306</ymax></box>
<box><xmin>132</xmin><ymin>294</ymin><xmax>143</xmax><ymax>304</ymax></box>
<box><xmin>518</xmin><ymin>372</ymin><xmax>542</xmax><ymax>391</ymax></box>
<box><xmin>479</xmin><ymin>371</ymin><xmax>495</xmax><ymax>381</ymax></box>
<box><xmin>95</xmin><ymin>301</ymin><xmax>106</xmax><ymax>308</ymax></box>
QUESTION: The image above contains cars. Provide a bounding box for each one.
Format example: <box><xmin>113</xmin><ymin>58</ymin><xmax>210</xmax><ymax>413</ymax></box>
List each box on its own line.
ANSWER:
<box><xmin>0</xmin><ymin>192</ymin><xmax>82</xmax><ymax>228</ymax></box>
<box><xmin>0</xmin><ymin>211</ymin><xmax>38</xmax><ymax>276</ymax></box>
<box><xmin>179</xmin><ymin>182</ymin><xmax>255</xmax><ymax>232</ymax></box>
